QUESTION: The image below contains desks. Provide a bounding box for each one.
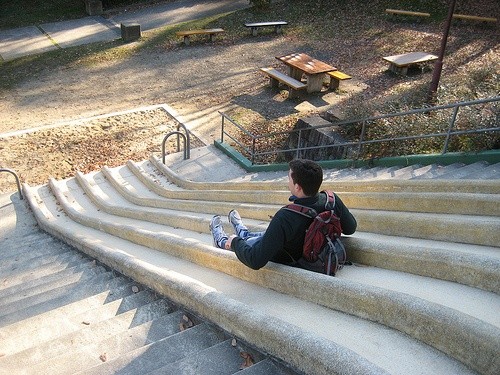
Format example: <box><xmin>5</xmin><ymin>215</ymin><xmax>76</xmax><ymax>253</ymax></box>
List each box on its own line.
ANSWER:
<box><xmin>274</xmin><ymin>51</ymin><xmax>337</xmax><ymax>93</ymax></box>
<box><xmin>382</xmin><ymin>51</ymin><xmax>439</xmax><ymax>76</ymax></box>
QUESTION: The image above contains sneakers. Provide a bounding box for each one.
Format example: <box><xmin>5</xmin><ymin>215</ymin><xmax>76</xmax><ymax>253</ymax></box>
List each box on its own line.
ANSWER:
<box><xmin>228</xmin><ymin>209</ymin><xmax>249</xmax><ymax>235</ymax></box>
<box><xmin>209</xmin><ymin>214</ymin><xmax>228</xmax><ymax>249</ymax></box>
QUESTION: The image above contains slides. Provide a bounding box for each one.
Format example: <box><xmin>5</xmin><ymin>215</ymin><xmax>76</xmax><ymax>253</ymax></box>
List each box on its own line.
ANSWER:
<box><xmin>21</xmin><ymin>155</ymin><xmax>500</xmax><ymax>375</ymax></box>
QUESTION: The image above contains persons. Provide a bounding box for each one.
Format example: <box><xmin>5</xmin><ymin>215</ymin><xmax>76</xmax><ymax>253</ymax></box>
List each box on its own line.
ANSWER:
<box><xmin>210</xmin><ymin>157</ymin><xmax>357</xmax><ymax>271</ymax></box>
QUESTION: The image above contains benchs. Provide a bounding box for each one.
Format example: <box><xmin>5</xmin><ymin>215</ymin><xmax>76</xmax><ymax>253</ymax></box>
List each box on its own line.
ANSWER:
<box><xmin>260</xmin><ymin>67</ymin><xmax>308</xmax><ymax>99</ymax></box>
<box><xmin>174</xmin><ymin>28</ymin><xmax>226</xmax><ymax>46</ymax></box>
<box><xmin>243</xmin><ymin>20</ymin><xmax>288</xmax><ymax>37</ymax></box>
<box><xmin>452</xmin><ymin>14</ymin><xmax>497</xmax><ymax>27</ymax></box>
<box><xmin>326</xmin><ymin>70</ymin><xmax>352</xmax><ymax>90</ymax></box>
<box><xmin>385</xmin><ymin>9</ymin><xmax>431</xmax><ymax>23</ymax></box>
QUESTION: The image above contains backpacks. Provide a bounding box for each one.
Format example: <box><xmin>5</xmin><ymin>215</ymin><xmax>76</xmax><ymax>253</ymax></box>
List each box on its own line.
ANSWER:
<box><xmin>283</xmin><ymin>190</ymin><xmax>347</xmax><ymax>277</ymax></box>
